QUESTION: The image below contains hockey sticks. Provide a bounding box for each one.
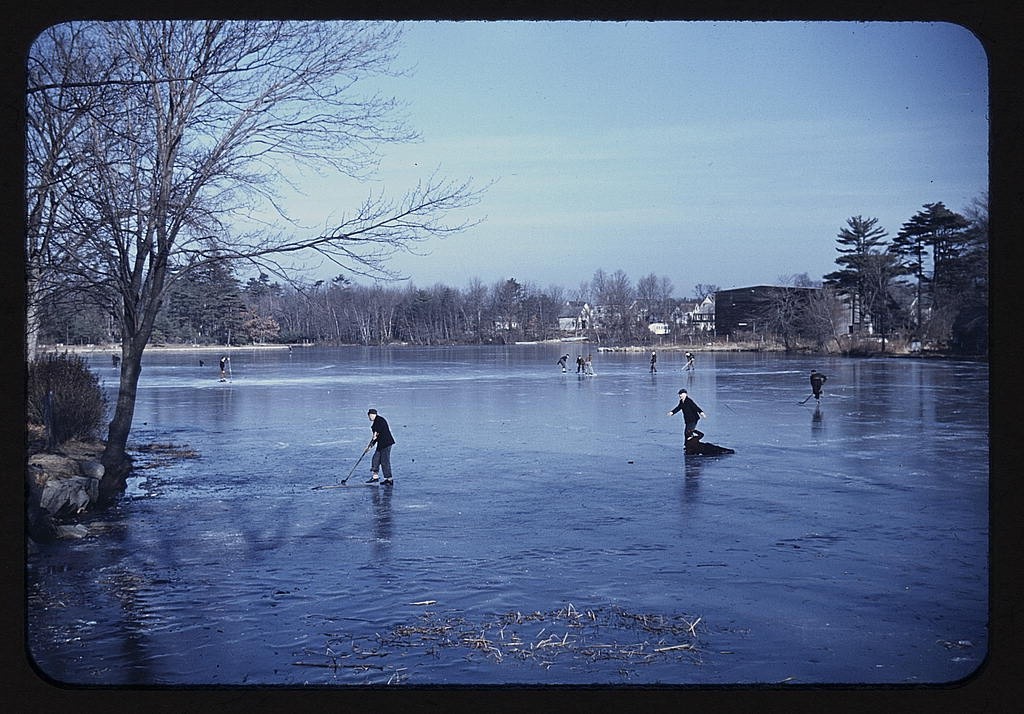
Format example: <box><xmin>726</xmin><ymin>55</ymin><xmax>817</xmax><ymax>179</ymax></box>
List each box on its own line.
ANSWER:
<box><xmin>565</xmin><ymin>363</ymin><xmax>571</xmax><ymax>371</ymax></box>
<box><xmin>681</xmin><ymin>360</ymin><xmax>691</xmax><ymax>370</ymax></box>
<box><xmin>575</xmin><ymin>363</ymin><xmax>578</xmax><ymax>373</ymax></box>
<box><xmin>341</xmin><ymin>434</ymin><xmax>377</xmax><ymax>484</ymax></box>
<box><xmin>798</xmin><ymin>393</ymin><xmax>813</xmax><ymax>404</ymax></box>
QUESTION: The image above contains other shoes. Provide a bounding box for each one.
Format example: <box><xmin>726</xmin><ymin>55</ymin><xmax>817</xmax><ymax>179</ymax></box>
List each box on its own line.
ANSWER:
<box><xmin>366</xmin><ymin>478</ymin><xmax>379</xmax><ymax>483</ymax></box>
<box><xmin>380</xmin><ymin>479</ymin><xmax>393</xmax><ymax>485</ymax></box>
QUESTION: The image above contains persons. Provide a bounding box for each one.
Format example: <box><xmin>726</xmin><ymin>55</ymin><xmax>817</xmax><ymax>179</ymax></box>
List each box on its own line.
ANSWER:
<box><xmin>668</xmin><ymin>389</ymin><xmax>707</xmax><ymax>449</ymax></box>
<box><xmin>684</xmin><ymin>429</ymin><xmax>735</xmax><ymax>455</ymax></box>
<box><xmin>685</xmin><ymin>351</ymin><xmax>695</xmax><ymax>371</ymax></box>
<box><xmin>55</xmin><ymin>347</ymin><xmax>59</xmax><ymax>353</ymax></box>
<box><xmin>44</xmin><ymin>347</ymin><xmax>47</xmax><ymax>351</ymax></box>
<box><xmin>576</xmin><ymin>354</ymin><xmax>592</xmax><ymax>374</ymax></box>
<box><xmin>220</xmin><ymin>356</ymin><xmax>230</xmax><ymax>381</ymax></box>
<box><xmin>365</xmin><ymin>409</ymin><xmax>395</xmax><ymax>486</ymax></box>
<box><xmin>649</xmin><ymin>350</ymin><xmax>657</xmax><ymax>373</ymax></box>
<box><xmin>559</xmin><ymin>354</ymin><xmax>572</xmax><ymax>373</ymax></box>
<box><xmin>112</xmin><ymin>353</ymin><xmax>120</xmax><ymax>369</ymax></box>
<box><xmin>289</xmin><ymin>345</ymin><xmax>293</xmax><ymax>352</ymax></box>
<box><xmin>810</xmin><ymin>369</ymin><xmax>827</xmax><ymax>404</ymax></box>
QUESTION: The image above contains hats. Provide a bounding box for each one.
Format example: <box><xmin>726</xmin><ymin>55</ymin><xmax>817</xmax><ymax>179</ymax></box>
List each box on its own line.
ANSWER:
<box><xmin>678</xmin><ymin>388</ymin><xmax>687</xmax><ymax>394</ymax></box>
<box><xmin>367</xmin><ymin>409</ymin><xmax>377</xmax><ymax>415</ymax></box>
